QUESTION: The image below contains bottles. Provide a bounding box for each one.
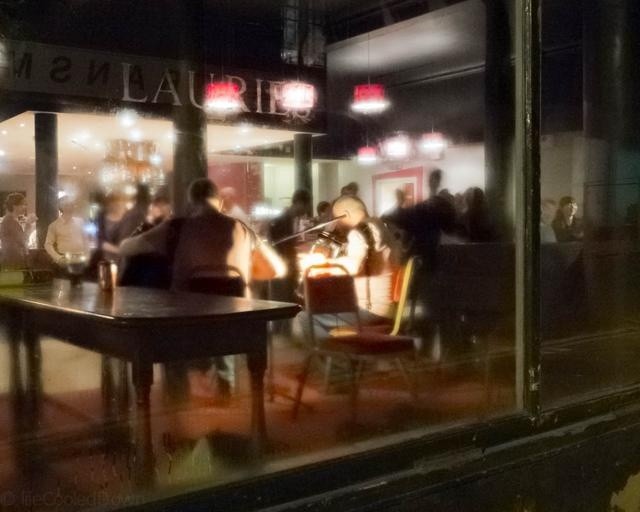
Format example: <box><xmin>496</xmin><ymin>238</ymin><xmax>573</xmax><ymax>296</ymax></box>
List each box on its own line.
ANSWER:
<box><xmin>98</xmin><ymin>261</ymin><xmax>117</xmax><ymax>292</ymax></box>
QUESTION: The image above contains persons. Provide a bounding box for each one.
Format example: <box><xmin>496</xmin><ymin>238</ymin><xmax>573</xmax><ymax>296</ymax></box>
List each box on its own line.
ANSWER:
<box><xmin>115</xmin><ymin>179</ymin><xmax>290</xmax><ymax>408</ymax></box>
<box><xmin>293</xmin><ymin>194</ymin><xmax>404</xmax><ymax>382</ymax></box>
<box><xmin>0</xmin><ymin>193</ymin><xmax>39</xmax><ymax>260</ymax></box>
<box><xmin>45</xmin><ymin>193</ymin><xmax>93</xmax><ymax>273</ymax></box>
<box><xmin>94</xmin><ymin>163</ymin><xmax>583</xmax><ymax>347</ymax></box>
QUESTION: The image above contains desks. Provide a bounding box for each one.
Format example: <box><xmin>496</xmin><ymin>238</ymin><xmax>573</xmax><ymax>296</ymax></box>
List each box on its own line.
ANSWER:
<box><xmin>0</xmin><ymin>276</ymin><xmax>300</xmax><ymax>487</ymax></box>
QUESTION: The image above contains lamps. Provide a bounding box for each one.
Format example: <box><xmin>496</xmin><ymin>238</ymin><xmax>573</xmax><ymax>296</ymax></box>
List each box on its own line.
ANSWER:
<box><xmin>202</xmin><ymin>0</ymin><xmax>446</xmax><ymax>161</ymax></box>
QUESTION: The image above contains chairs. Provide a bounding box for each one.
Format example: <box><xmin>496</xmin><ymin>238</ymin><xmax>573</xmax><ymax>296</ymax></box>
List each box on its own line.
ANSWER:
<box><xmin>291</xmin><ymin>262</ymin><xmax>424</xmax><ymax>434</ymax></box>
<box><xmin>186</xmin><ymin>265</ymin><xmax>275</xmax><ymax>400</ymax></box>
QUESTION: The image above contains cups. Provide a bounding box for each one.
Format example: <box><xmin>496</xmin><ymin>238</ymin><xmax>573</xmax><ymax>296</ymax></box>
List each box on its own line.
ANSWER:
<box><xmin>64</xmin><ymin>252</ymin><xmax>85</xmax><ymax>290</ymax></box>
